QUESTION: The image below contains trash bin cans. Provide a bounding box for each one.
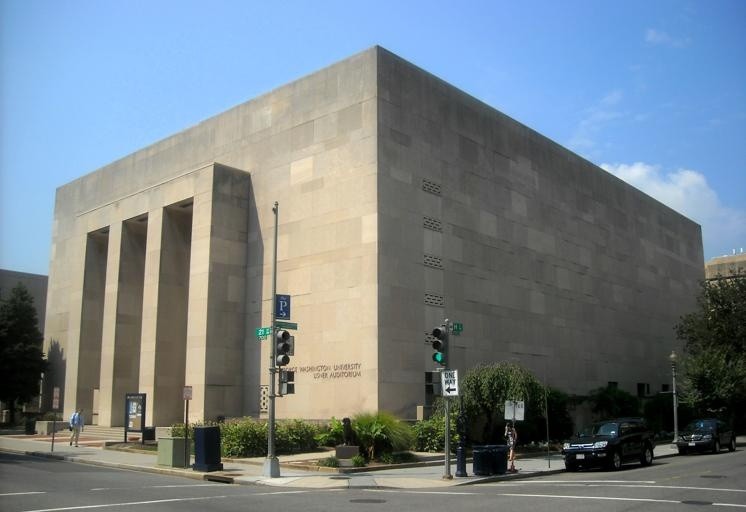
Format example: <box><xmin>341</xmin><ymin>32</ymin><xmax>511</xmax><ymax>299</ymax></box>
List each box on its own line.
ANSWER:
<box><xmin>472</xmin><ymin>445</ymin><xmax>490</xmax><ymax>474</ymax></box>
<box><xmin>488</xmin><ymin>445</ymin><xmax>509</xmax><ymax>474</ymax></box>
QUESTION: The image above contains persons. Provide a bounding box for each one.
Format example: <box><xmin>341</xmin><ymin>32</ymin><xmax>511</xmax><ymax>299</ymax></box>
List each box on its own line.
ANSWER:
<box><xmin>504</xmin><ymin>422</ymin><xmax>517</xmax><ymax>461</ymax></box>
<box><xmin>69</xmin><ymin>407</ymin><xmax>84</xmax><ymax>447</ymax></box>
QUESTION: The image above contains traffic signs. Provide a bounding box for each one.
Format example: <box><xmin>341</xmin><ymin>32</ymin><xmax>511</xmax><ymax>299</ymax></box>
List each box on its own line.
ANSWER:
<box><xmin>440</xmin><ymin>370</ymin><xmax>459</xmax><ymax>397</ymax></box>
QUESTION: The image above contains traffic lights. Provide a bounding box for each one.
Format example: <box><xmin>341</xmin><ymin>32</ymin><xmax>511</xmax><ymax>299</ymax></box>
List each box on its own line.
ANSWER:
<box><xmin>432</xmin><ymin>325</ymin><xmax>448</xmax><ymax>366</ymax></box>
<box><xmin>274</xmin><ymin>328</ymin><xmax>290</xmax><ymax>366</ymax></box>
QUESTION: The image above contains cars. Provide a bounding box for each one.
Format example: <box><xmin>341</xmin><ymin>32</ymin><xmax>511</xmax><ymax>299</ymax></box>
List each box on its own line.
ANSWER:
<box><xmin>561</xmin><ymin>418</ymin><xmax>736</xmax><ymax>473</ymax></box>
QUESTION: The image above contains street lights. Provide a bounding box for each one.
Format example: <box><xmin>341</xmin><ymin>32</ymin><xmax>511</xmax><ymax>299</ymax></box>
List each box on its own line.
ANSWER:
<box><xmin>669</xmin><ymin>351</ymin><xmax>681</xmax><ymax>443</ymax></box>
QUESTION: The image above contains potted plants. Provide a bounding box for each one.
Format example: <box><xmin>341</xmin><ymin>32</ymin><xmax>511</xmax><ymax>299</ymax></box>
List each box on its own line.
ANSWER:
<box><xmin>348</xmin><ymin>411</ymin><xmax>418</xmax><ymax>461</ymax></box>
